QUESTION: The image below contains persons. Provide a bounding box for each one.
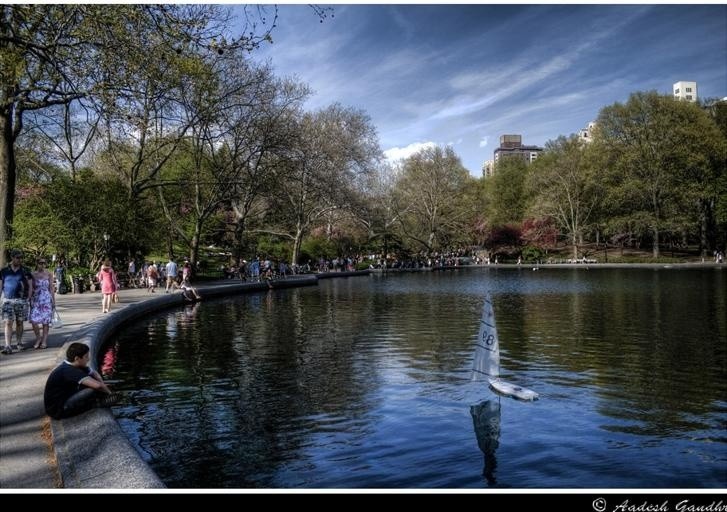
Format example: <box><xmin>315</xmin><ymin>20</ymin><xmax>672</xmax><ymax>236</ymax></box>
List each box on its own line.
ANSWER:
<box><xmin>0</xmin><ymin>249</ymin><xmax>33</xmax><ymax>354</ymax></box>
<box><xmin>53</xmin><ymin>262</ymin><xmax>66</xmax><ymax>295</ymax></box>
<box><xmin>226</xmin><ymin>257</ymin><xmax>356</xmax><ymax>283</ymax></box>
<box><xmin>96</xmin><ymin>257</ymin><xmax>117</xmax><ymax>310</ymax></box>
<box><xmin>99</xmin><ymin>260</ymin><xmax>119</xmax><ymax>313</ymax></box>
<box><xmin>43</xmin><ymin>342</ymin><xmax>122</xmax><ymax>419</ymax></box>
<box><xmin>127</xmin><ymin>256</ymin><xmax>202</xmax><ymax>300</ymax></box>
<box><xmin>28</xmin><ymin>257</ymin><xmax>57</xmax><ymax>349</ymax></box>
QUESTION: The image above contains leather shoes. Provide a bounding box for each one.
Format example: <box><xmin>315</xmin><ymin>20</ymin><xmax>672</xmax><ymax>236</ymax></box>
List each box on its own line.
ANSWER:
<box><xmin>33</xmin><ymin>338</ymin><xmax>48</xmax><ymax>349</ymax></box>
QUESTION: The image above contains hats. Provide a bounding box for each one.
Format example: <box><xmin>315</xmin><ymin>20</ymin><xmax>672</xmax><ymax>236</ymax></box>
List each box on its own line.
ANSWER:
<box><xmin>10</xmin><ymin>250</ymin><xmax>23</xmax><ymax>258</ymax></box>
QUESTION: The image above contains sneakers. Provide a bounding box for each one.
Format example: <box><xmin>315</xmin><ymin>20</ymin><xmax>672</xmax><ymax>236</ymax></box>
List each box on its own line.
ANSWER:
<box><xmin>1</xmin><ymin>342</ymin><xmax>25</xmax><ymax>356</ymax></box>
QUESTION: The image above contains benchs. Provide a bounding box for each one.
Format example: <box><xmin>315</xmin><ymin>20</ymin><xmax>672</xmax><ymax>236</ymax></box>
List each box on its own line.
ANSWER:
<box><xmin>85</xmin><ymin>271</ymin><xmax>138</xmax><ymax>291</ymax></box>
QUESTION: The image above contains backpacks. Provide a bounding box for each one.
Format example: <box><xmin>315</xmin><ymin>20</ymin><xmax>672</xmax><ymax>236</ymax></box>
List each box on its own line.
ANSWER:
<box><xmin>4</xmin><ymin>274</ymin><xmax>23</xmax><ymax>300</ymax></box>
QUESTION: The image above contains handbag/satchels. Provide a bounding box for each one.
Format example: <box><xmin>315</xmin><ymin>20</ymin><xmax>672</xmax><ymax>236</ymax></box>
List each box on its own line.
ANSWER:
<box><xmin>112</xmin><ymin>292</ymin><xmax>120</xmax><ymax>303</ymax></box>
<box><xmin>51</xmin><ymin>317</ymin><xmax>63</xmax><ymax>330</ymax></box>
